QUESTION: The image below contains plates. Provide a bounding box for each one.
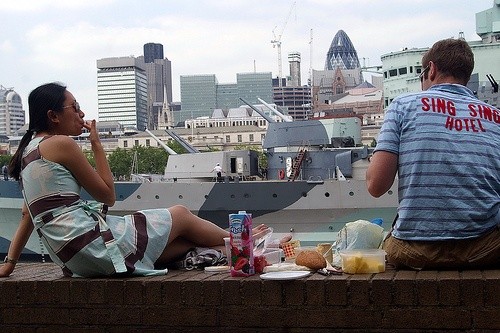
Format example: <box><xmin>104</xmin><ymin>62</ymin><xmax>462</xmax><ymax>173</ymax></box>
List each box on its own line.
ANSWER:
<box><xmin>259</xmin><ymin>271</ymin><xmax>310</xmax><ymax>280</ymax></box>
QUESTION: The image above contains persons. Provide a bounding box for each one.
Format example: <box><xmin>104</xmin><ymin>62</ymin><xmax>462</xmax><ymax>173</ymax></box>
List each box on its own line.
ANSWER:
<box><xmin>0</xmin><ymin>83</ymin><xmax>292</xmax><ymax>278</ymax></box>
<box><xmin>210</xmin><ymin>163</ymin><xmax>225</xmax><ymax>182</ymax></box>
<box><xmin>366</xmin><ymin>38</ymin><xmax>500</xmax><ymax>271</ymax></box>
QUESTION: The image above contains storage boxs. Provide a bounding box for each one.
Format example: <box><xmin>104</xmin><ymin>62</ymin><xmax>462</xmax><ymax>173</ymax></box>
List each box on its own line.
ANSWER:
<box><xmin>338</xmin><ymin>248</ymin><xmax>387</xmax><ymax>274</ymax></box>
<box><xmin>223</xmin><ymin>235</ymin><xmax>283</xmax><ymax>265</ymax></box>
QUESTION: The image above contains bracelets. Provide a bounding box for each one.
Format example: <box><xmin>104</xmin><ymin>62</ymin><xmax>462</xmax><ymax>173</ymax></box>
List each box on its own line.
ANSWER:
<box><xmin>3</xmin><ymin>256</ymin><xmax>18</xmax><ymax>265</ymax></box>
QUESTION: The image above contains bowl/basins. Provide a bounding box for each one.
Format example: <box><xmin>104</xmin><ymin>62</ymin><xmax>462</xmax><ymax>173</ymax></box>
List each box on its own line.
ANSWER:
<box><xmin>340</xmin><ymin>249</ymin><xmax>387</xmax><ymax>273</ymax></box>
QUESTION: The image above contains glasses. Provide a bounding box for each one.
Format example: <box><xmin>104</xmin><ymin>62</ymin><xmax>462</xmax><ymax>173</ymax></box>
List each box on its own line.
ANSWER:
<box><xmin>51</xmin><ymin>102</ymin><xmax>80</xmax><ymax>112</ymax></box>
<box><xmin>419</xmin><ymin>61</ymin><xmax>437</xmax><ymax>83</ymax></box>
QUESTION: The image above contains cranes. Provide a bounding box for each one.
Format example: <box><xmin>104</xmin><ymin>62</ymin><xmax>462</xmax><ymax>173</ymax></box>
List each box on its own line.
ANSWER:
<box><xmin>270</xmin><ymin>2</ymin><xmax>295</xmax><ymax>87</ymax></box>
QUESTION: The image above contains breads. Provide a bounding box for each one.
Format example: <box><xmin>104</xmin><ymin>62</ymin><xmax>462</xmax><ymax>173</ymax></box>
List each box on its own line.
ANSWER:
<box><xmin>295</xmin><ymin>249</ymin><xmax>327</xmax><ymax>269</ymax></box>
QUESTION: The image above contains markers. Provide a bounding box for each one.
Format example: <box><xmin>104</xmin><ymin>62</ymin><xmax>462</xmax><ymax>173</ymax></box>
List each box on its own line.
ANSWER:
<box><xmin>204</xmin><ymin>265</ymin><xmax>230</xmax><ymax>271</ymax></box>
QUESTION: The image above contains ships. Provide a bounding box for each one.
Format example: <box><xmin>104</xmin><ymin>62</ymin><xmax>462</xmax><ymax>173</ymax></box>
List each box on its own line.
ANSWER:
<box><xmin>0</xmin><ymin>3</ymin><xmax>500</xmax><ymax>259</ymax></box>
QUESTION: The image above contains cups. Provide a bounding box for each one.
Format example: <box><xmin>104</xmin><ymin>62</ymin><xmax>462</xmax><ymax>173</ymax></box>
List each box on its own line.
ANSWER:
<box><xmin>223</xmin><ymin>238</ymin><xmax>230</xmax><ymax>266</ymax></box>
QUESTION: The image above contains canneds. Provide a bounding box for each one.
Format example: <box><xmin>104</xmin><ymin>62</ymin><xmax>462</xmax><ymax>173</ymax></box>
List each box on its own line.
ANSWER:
<box><xmin>317</xmin><ymin>243</ymin><xmax>333</xmax><ymax>264</ymax></box>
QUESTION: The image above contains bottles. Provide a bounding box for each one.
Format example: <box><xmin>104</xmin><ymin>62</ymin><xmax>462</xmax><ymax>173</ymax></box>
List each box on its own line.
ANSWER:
<box><xmin>316</xmin><ymin>243</ymin><xmax>333</xmax><ymax>263</ymax></box>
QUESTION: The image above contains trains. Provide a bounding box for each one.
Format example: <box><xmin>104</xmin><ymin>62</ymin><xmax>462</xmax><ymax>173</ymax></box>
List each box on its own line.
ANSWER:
<box><xmin>1</xmin><ymin>121</ymin><xmax>125</xmax><ymax>140</ymax></box>
